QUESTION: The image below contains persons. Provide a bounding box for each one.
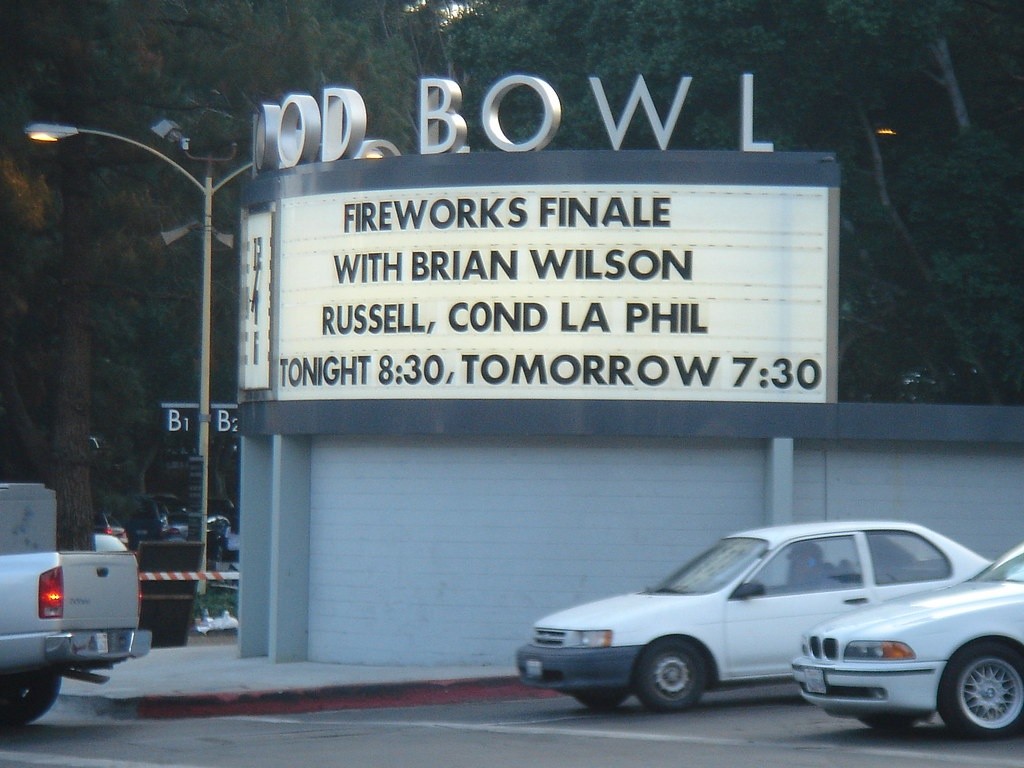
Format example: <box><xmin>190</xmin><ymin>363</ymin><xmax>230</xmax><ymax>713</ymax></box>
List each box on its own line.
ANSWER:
<box><xmin>788</xmin><ymin>542</ymin><xmax>839</xmax><ymax>591</ymax></box>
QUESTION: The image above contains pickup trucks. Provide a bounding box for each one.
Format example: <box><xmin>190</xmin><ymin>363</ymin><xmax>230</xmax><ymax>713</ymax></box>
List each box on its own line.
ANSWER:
<box><xmin>0</xmin><ymin>482</ymin><xmax>152</xmax><ymax>730</ymax></box>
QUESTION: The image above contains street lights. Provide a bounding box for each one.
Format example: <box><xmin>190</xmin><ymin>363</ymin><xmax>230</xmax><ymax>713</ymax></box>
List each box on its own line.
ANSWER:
<box><xmin>22</xmin><ymin>122</ymin><xmax>385</xmax><ymax>598</ymax></box>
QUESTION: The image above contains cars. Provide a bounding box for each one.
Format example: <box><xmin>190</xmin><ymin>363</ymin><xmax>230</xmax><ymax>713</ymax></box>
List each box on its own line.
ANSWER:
<box><xmin>789</xmin><ymin>541</ymin><xmax>1024</xmax><ymax>742</ymax></box>
<box><xmin>96</xmin><ymin>493</ymin><xmax>229</xmax><ymax>551</ymax></box>
<box><xmin>517</xmin><ymin>518</ymin><xmax>1022</xmax><ymax>717</ymax></box>
